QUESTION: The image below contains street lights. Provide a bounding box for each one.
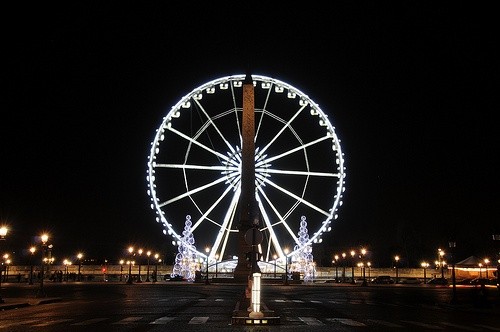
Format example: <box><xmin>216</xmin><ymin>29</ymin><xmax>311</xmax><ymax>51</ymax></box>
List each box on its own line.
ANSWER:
<box><xmin>350</xmin><ymin>250</ymin><xmax>356</xmax><ymax>284</ymax></box>
<box><xmin>77</xmin><ymin>252</ymin><xmax>81</xmax><ymax>282</ymax></box>
<box><xmin>102</xmin><ymin>246</ymin><xmax>162</xmax><ymax>283</ymax></box>
<box><xmin>478</xmin><ymin>263</ymin><xmax>483</xmax><ymax>277</ymax></box>
<box><xmin>395</xmin><ymin>255</ymin><xmax>400</xmax><ymax>283</ymax></box>
<box><xmin>341</xmin><ymin>252</ymin><xmax>347</xmax><ymax>282</ymax></box>
<box><xmin>334</xmin><ymin>254</ymin><xmax>339</xmax><ymax>283</ymax></box>
<box><xmin>367</xmin><ymin>261</ymin><xmax>371</xmax><ymax>282</ymax></box>
<box><xmin>357</xmin><ymin>262</ymin><xmax>364</xmax><ymax>280</ymax></box>
<box><xmin>438</xmin><ymin>248</ymin><xmax>446</xmax><ymax>278</ymax></box>
<box><xmin>448</xmin><ymin>241</ymin><xmax>456</xmax><ymax>286</ymax></box>
<box><xmin>361</xmin><ymin>248</ymin><xmax>368</xmax><ymax>286</ymax></box>
<box><xmin>421</xmin><ymin>261</ymin><xmax>430</xmax><ymax>284</ymax></box>
<box><xmin>215</xmin><ymin>253</ymin><xmax>220</xmax><ymax>277</ymax></box>
<box><xmin>0</xmin><ymin>225</ymin><xmax>73</xmax><ymax>287</ymax></box>
<box><xmin>205</xmin><ymin>247</ymin><xmax>210</xmax><ymax>281</ymax></box>
<box><xmin>283</xmin><ymin>248</ymin><xmax>289</xmax><ymax>285</ymax></box>
<box><xmin>484</xmin><ymin>258</ymin><xmax>490</xmax><ymax>278</ymax></box>
<box><xmin>273</xmin><ymin>254</ymin><xmax>277</xmax><ymax>278</ymax></box>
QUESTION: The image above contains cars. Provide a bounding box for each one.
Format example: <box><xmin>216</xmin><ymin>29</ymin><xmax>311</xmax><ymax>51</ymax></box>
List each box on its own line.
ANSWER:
<box><xmin>426</xmin><ymin>277</ymin><xmax>448</xmax><ymax>285</ymax></box>
<box><xmin>400</xmin><ymin>277</ymin><xmax>420</xmax><ymax>284</ymax></box>
<box><xmin>371</xmin><ymin>275</ymin><xmax>392</xmax><ymax>285</ymax></box>
<box><xmin>164</xmin><ymin>272</ymin><xmax>186</xmax><ymax>282</ymax></box>
<box><xmin>461</xmin><ymin>276</ymin><xmax>495</xmax><ymax>287</ymax></box>
